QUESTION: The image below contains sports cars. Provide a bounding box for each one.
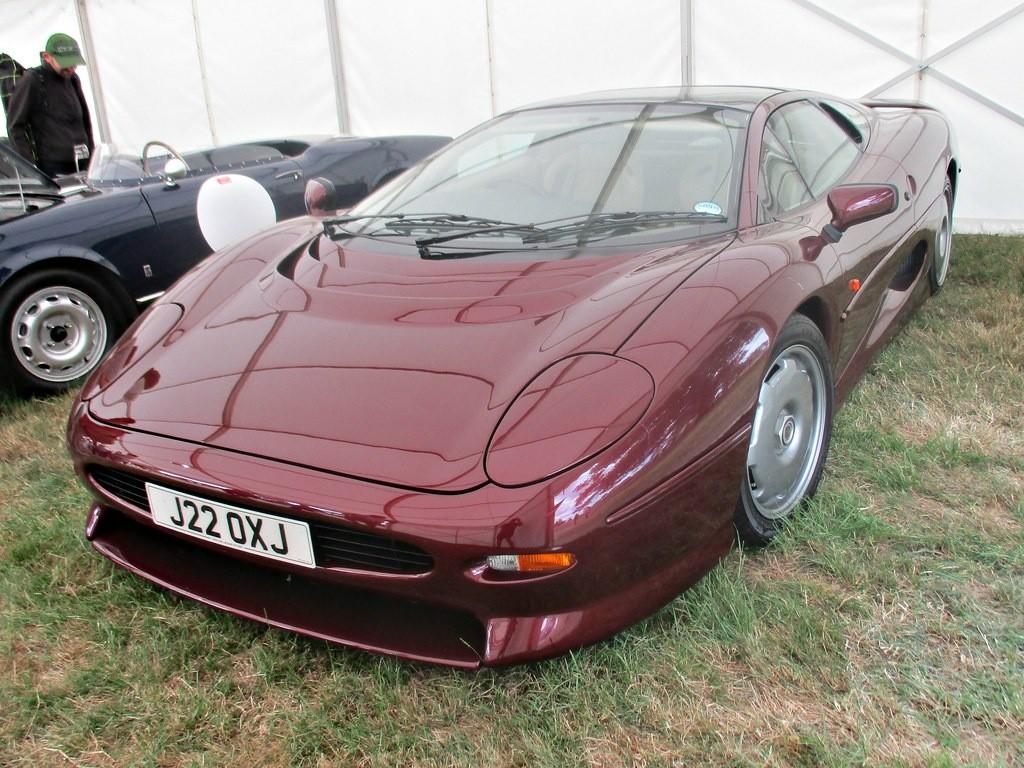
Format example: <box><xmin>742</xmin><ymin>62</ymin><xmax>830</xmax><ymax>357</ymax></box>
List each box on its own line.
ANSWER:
<box><xmin>0</xmin><ymin>133</ymin><xmax>457</xmax><ymax>394</ymax></box>
<box><xmin>66</xmin><ymin>86</ymin><xmax>959</xmax><ymax>677</ymax></box>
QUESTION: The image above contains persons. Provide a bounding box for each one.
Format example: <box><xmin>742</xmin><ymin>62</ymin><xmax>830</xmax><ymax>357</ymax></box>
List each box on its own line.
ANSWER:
<box><xmin>5</xmin><ymin>32</ymin><xmax>97</xmax><ymax>179</ymax></box>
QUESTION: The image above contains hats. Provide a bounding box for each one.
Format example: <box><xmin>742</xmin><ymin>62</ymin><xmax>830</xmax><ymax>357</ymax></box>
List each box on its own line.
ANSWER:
<box><xmin>46</xmin><ymin>34</ymin><xmax>87</xmax><ymax>68</ymax></box>
